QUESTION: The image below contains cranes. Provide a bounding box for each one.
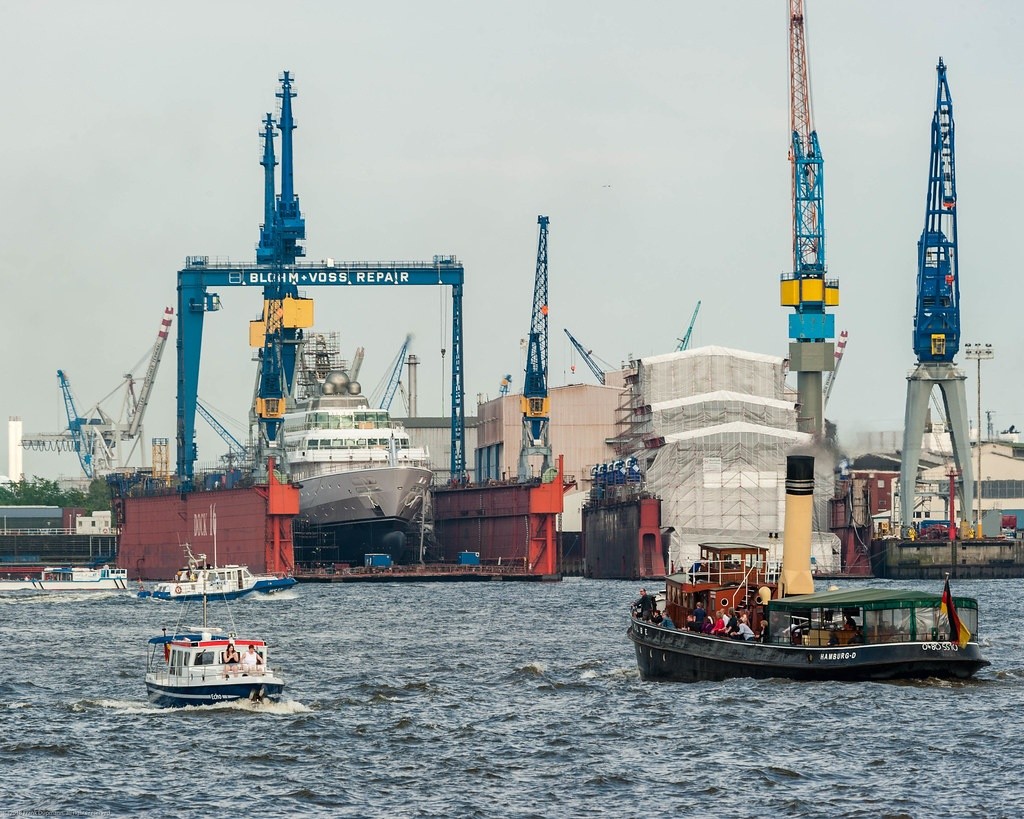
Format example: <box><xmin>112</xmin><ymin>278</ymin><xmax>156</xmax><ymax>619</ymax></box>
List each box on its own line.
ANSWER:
<box><xmin>368</xmin><ymin>335</ymin><xmax>411</xmax><ymax>411</ymax></box>
<box><xmin>780</xmin><ymin>0</ymin><xmax>841</xmax><ymax>433</ymax></box>
<box><xmin>564</xmin><ymin>328</ymin><xmax>606</xmax><ymax>385</ymax></box>
<box><xmin>518</xmin><ymin>214</ymin><xmax>553</xmax><ymax>479</ymax></box>
<box><xmin>196</xmin><ymin>395</ymin><xmax>249</xmax><ymax>460</ymax></box>
<box><xmin>674</xmin><ymin>301</ymin><xmax>701</xmax><ymax>352</ymax></box>
<box><xmin>897</xmin><ymin>57</ymin><xmax>975</xmax><ymax>544</ymax></box>
<box><xmin>398</xmin><ymin>381</ymin><xmax>409</xmax><ymax>417</ymax></box>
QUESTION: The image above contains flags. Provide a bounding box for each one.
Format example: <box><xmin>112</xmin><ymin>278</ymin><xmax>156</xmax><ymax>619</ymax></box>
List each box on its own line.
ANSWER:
<box><xmin>940</xmin><ymin>580</ymin><xmax>971</xmax><ymax>649</ymax></box>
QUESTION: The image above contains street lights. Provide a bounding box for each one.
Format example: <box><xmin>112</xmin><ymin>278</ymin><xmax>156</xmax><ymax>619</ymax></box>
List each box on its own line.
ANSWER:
<box><xmin>966</xmin><ymin>344</ymin><xmax>993</xmax><ymax>541</ymax></box>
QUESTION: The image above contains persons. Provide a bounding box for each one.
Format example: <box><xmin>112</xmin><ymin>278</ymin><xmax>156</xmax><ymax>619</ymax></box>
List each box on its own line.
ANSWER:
<box><xmin>223</xmin><ymin>644</ymin><xmax>239</xmax><ymax>679</ymax></box>
<box><xmin>631</xmin><ymin>557</ymin><xmax>770</xmax><ymax>644</ymax></box>
<box><xmin>846</xmin><ymin>615</ymin><xmax>857</xmax><ymax>628</ymax></box>
<box><xmin>242</xmin><ymin>644</ymin><xmax>264</xmax><ymax>675</ymax></box>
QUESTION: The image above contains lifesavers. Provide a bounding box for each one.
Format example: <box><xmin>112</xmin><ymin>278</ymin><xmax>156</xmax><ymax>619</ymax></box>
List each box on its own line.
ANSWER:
<box><xmin>175</xmin><ymin>586</ymin><xmax>182</xmax><ymax>594</ymax></box>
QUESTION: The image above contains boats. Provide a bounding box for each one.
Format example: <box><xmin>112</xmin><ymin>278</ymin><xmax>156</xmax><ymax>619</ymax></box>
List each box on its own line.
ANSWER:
<box><xmin>0</xmin><ymin>565</ymin><xmax>128</xmax><ymax>589</ymax></box>
<box><xmin>152</xmin><ymin>534</ymin><xmax>300</xmax><ymax>602</ymax></box>
<box><xmin>248</xmin><ymin>330</ymin><xmax>434</xmax><ymax>529</ymax></box>
<box><xmin>625</xmin><ymin>459</ymin><xmax>992</xmax><ymax>684</ymax></box>
<box><xmin>145</xmin><ymin>595</ymin><xmax>285</xmax><ymax>708</ymax></box>
